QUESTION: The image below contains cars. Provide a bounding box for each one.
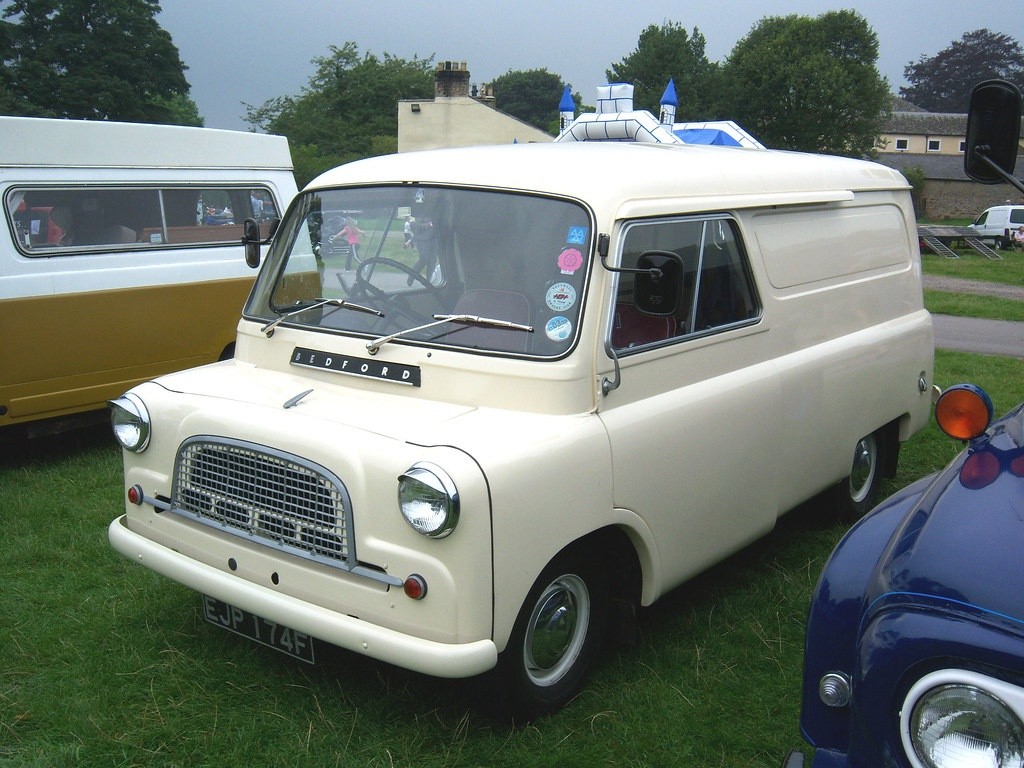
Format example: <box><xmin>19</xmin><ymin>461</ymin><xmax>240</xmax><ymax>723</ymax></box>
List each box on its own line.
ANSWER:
<box><xmin>309</xmin><ymin>227</ymin><xmax>351</xmax><ymax>257</ymax></box>
<box><xmin>782</xmin><ymin>79</ymin><xmax>1024</xmax><ymax>768</ymax></box>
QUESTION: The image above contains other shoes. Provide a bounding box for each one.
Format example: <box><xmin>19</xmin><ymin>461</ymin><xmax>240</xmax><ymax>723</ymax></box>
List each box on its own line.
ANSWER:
<box><xmin>364</xmin><ymin>265</ymin><xmax>369</xmax><ymax>271</ymax></box>
<box><xmin>407</xmin><ymin>277</ymin><xmax>414</xmax><ymax>286</ymax></box>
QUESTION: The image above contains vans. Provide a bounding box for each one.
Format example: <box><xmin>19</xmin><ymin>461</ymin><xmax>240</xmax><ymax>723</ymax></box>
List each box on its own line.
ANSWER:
<box><xmin>108</xmin><ymin>140</ymin><xmax>936</xmax><ymax>716</ymax></box>
<box><xmin>0</xmin><ymin>115</ymin><xmax>321</xmax><ymax>428</ymax></box>
<box><xmin>967</xmin><ymin>205</ymin><xmax>1024</xmax><ymax>250</ymax></box>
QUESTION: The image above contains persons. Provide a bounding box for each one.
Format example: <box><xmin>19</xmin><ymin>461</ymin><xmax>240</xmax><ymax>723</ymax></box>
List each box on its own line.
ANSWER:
<box><xmin>407</xmin><ymin>213</ymin><xmax>439</xmax><ymax>287</ymax></box>
<box><xmin>333</xmin><ymin>216</ymin><xmax>369</xmax><ymax>274</ymax></box>
<box><xmin>196</xmin><ymin>189</ymin><xmax>274</xmax><ymax>228</ymax></box>
<box><xmin>50</xmin><ymin>206</ymin><xmax>81</xmax><ymax>247</ymax></box>
<box><xmin>403</xmin><ymin>217</ymin><xmax>414</xmax><ymax>249</ymax></box>
<box><xmin>1013</xmin><ymin>225</ymin><xmax>1024</xmax><ymax>253</ymax></box>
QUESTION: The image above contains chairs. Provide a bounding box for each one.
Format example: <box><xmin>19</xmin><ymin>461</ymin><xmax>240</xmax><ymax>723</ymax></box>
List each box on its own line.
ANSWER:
<box><xmin>444</xmin><ymin>289</ymin><xmax>536</xmax><ymax>352</ymax></box>
